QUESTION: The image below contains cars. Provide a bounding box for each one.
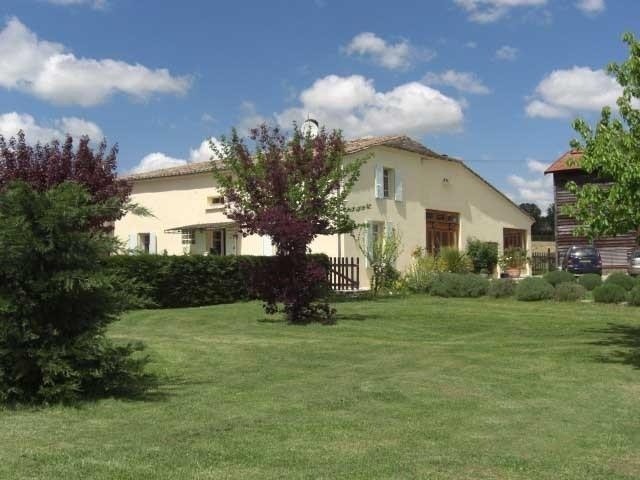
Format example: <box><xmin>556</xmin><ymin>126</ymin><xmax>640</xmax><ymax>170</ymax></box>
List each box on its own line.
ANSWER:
<box><xmin>628</xmin><ymin>252</ymin><xmax>640</xmax><ymax>275</ymax></box>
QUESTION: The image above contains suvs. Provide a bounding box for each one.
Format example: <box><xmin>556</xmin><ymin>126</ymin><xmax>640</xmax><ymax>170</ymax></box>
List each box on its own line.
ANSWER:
<box><xmin>564</xmin><ymin>244</ymin><xmax>603</xmax><ymax>279</ymax></box>
